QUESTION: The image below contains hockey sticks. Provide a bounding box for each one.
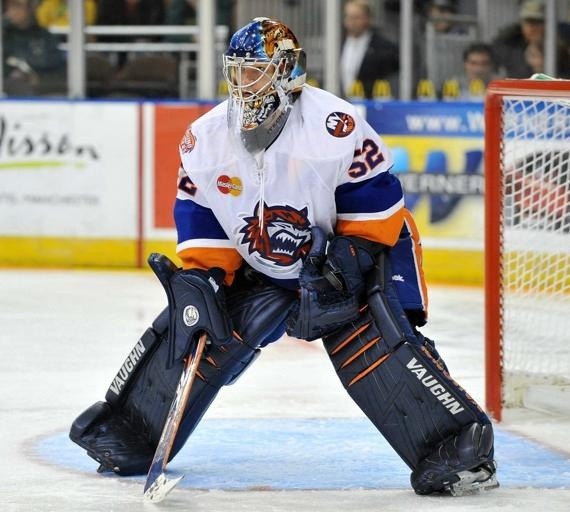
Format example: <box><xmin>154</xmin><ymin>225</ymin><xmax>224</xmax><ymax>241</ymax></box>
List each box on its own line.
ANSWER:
<box><xmin>143</xmin><ymin>277</ymin><xmax>219</xmax><ymax>504</ymax></box>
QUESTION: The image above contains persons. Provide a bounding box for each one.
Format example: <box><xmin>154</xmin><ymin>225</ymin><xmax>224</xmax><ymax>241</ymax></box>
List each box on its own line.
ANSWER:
<box><xmin>489</xmin><ymin>0</ymin><xmax>546</xmax><ymax>80</ymax></box>
<box><xmin>450</xmin><ymin>43</ymin><xmax>503</xmax><ymax>93</ymax></box>
<box><xmin>68</xmin><ymin>12</ymin><xmax>496</xmax><ymax>497</ymax></box>
<box><xmin>338</xmin><ymin>3</ymin><xmax>398</xmax><ymax>102</ymax></box>
<box><xmin>1</xmin><ymin>0</ymin><xmax>62</xmax><ymax>98</ymax></box>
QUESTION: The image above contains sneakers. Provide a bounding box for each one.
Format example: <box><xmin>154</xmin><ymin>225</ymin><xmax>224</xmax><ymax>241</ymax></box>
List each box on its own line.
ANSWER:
<box><xmin>444</xmin><ymin>455</ymin><xmax>496</xmax><ymax>488</ymax></box>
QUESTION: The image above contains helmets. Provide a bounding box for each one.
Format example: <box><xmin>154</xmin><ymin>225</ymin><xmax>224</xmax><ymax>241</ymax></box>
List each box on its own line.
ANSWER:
<box><xmin>222</xmin><ymin>15</ymin><xmax>308</xmax><ymax>148</ymax></box>
